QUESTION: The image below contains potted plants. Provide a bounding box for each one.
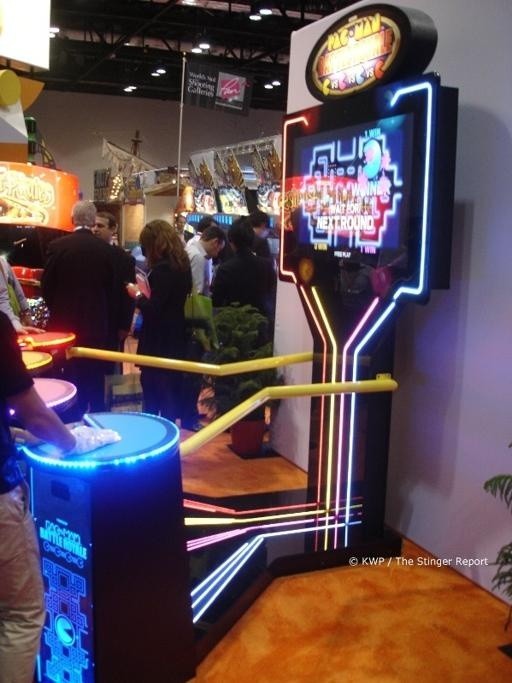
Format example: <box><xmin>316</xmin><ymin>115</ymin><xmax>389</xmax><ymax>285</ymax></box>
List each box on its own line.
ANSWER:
<box><xmin>202</xmin><ymin>304</ymin><xmax>284</xmax><ymax>455</ymax></box>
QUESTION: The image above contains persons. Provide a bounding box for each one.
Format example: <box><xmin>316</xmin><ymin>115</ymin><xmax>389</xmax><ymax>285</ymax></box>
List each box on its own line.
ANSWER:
<box><xmin>1</xmin><ymin>197</ymin><xmax>277</xmax><ymax>431</ymax></box>
<box><xmin>1</xmin><ymin>311</ymin><xmax>123</xmax><ymax>683</ymax></box>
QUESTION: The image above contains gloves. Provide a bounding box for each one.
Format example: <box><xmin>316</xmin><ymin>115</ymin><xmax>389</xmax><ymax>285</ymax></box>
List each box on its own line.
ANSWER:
<box><xmin>59</xmin><ymin>427</ymin><xmax>119</xmax><ymax>456</ymax></box>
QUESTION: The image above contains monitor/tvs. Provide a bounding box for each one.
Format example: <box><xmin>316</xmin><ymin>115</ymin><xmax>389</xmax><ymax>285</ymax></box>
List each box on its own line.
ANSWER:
<box><xmin>279</xmin><ymin>71</ymin><xmax>458</xmax><ymax>306</ymax></box>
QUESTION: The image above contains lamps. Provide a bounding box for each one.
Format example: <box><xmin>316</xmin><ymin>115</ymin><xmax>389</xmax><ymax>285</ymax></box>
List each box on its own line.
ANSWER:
<box><xmin>249</xmin><ymin>1</ymin><xmax>272</xmax><ymax>21</ymax></box>
<box><xmin>263</xmin><ymin>73</ymin><xmax>281</xmax><ymax>89</ymax></box>
<box><xmin>122</xmin><ymin>70</ymin><xmax>139</xmax><ymax>93</ymax></box>
<box><xmin>150</xmin><ymin>61</ymin><xmax>167</xmax><ymax>77</ymax></box>
<box><xmin>192</xmin><ymin>31</ymin><xmax>211</xmax><ymax>53</ymax></box>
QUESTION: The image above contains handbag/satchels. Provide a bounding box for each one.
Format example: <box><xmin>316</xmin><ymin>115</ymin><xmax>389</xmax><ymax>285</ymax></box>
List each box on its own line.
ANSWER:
<box><xmin>185</xmin><ymin>294</ymin><xmax>219</xmax><ymax>353</ymax></box>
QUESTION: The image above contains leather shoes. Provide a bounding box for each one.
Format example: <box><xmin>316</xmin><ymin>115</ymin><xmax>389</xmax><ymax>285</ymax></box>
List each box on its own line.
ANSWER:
<box><xmin>198</xmin><ymin>414</ymin><xmax>207</xmax><ymax>419</ymax></box>
<box><xmin>181</xmin><ymin>422</ymin><xmax>204</xmax><ymax>432</ymax></box>
